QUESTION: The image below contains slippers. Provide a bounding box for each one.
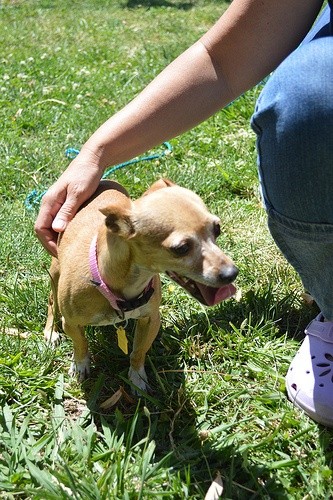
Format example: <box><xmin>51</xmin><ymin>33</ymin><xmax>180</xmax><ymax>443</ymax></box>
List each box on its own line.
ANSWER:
<box><xmin>284</xmin><ymin>311</ymin><xmax>333</xmax><ymax>431</ymax></box>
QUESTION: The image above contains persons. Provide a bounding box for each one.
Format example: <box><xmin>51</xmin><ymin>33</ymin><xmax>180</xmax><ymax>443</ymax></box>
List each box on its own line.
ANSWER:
<box><xmin>34</xmin><ymin>0</ymin><xmax>333</xmax><ymax>426</ymax></box>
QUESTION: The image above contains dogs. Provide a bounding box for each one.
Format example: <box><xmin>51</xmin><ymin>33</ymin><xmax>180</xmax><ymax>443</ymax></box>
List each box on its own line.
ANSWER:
<box><xmin>43</xmin><ymin>176</ymin><xmax>240</xmax><ymax>392</ymax></box>
